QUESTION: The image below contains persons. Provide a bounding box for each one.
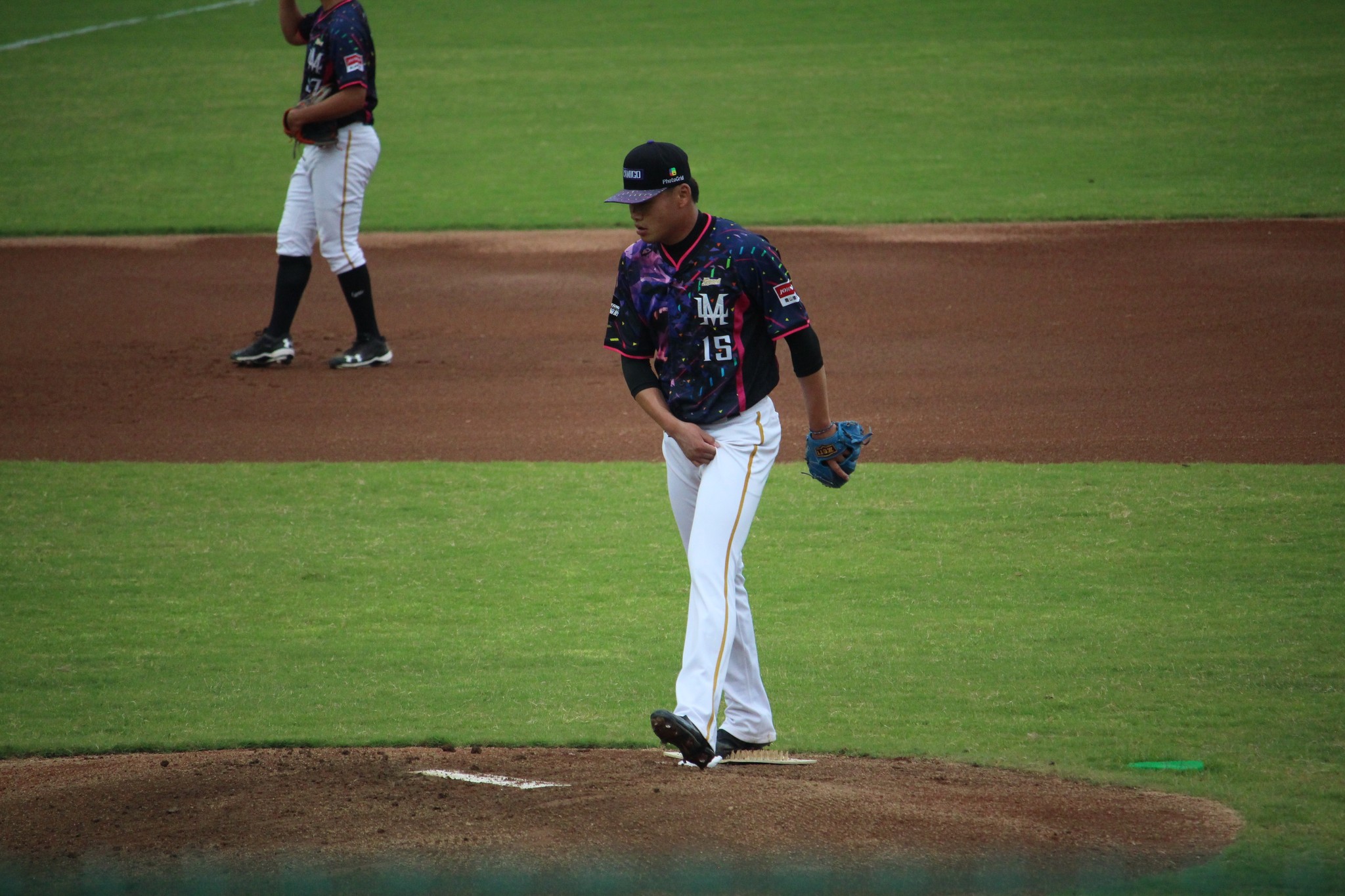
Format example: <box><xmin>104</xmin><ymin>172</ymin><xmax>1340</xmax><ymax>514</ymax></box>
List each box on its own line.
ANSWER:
<box><xmin>605</xmin><ymin>142</ymin><xmax>872</xmax><ymax>770</ymax></box>
<box><xmin>231</xmin><ymin>0</ymin><xmax>395</xmax><ymax>371</ymax></box>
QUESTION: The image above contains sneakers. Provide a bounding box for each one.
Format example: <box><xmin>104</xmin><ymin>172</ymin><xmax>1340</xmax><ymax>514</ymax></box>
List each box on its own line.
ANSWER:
<box><xmin>328</xmin><ymin>335</ymin><xmax>393</xmax><ymax>369</ymax></box>
<box><xmin>230</xmin><ymin>328</ymin><xmax>293</xmax><ymax>368</ymax></box>
<box><xmin>714</xmin><ymin>724</ymin><xmax>773</xmax><ymax>758</ymax></box>
<box><xmin>650</xmin><ymin>708</ymin><xmax>716</xmax><ymax>770</ymax></box>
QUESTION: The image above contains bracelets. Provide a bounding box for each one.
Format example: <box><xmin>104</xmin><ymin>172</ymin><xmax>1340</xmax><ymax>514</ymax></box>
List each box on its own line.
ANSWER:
<box><xmin>809</xmin><ymin>422</ymin><xmax>834</xmax><ymax>434</ymax></box>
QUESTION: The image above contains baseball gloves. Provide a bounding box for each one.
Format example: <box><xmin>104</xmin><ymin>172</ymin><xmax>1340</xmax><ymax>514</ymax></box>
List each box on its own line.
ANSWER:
<box><xmin>282</xmin><ymin>82</ymin><xmax>339</xmax><ymax>148</ymax></box>
<box><xmin>805</xmin><ymin>421</ymin><xmax>874</xmax><ymax>489</ymax></box>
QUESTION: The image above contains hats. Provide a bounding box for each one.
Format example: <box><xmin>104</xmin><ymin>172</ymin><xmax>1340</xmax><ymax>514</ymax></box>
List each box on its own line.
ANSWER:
<box><xmin>604</xmin><ymin>139</ymin><xmax>691</xmax><ymax>205</ymax></box>
